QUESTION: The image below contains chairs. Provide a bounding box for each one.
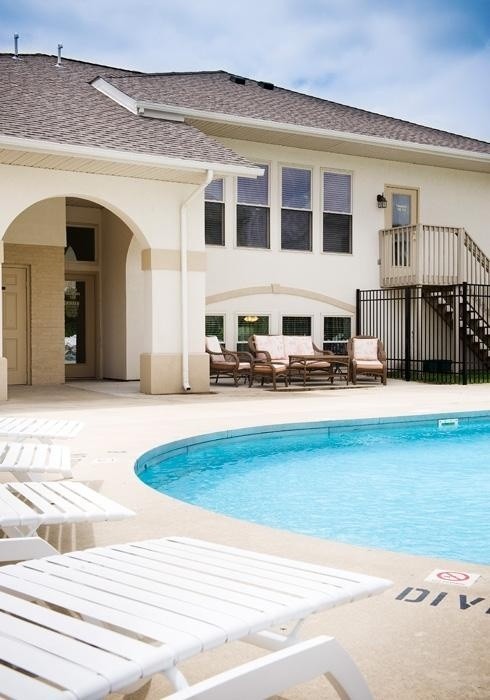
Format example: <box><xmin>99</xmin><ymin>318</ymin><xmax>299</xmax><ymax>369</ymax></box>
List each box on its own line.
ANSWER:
<box><xmin>0</xmin><ymin>411</ymin><xmax>81</xmax><ymax>442</ymax></box>
<box><xmin>1</xmin><ymin>533</ymin><xmax>394</xmax><ymax>699</ymax></box>
<box><xmin>204</xmin><ymin>334</ymin><xmax>389</xmax><ymax>390</ymax></box>
<box><xmin>0</xmin><ymin>441</ymin><xmax>76</xmax><ymax>476</ymax></box>
<box><xmin>1</xmin><ymin>479</ymin><xmax>138</xmax><ymax>564</ymax></box>
